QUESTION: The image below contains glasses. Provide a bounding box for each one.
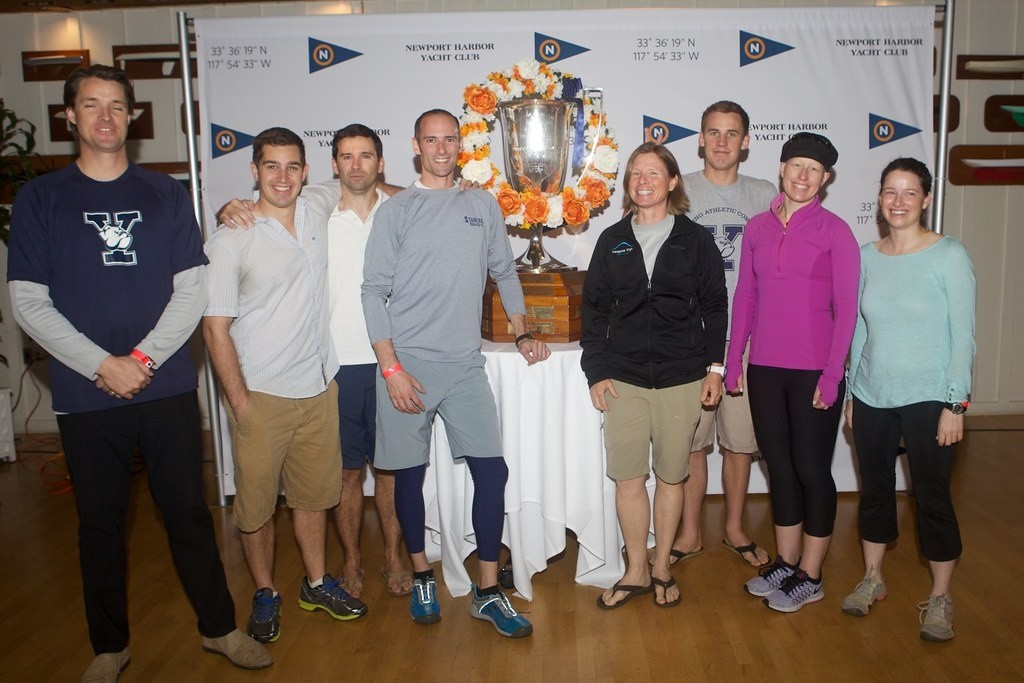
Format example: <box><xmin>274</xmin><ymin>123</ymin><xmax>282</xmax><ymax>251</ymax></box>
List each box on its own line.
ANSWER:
<box><xmin>792</xmin><ymin>132</ymin><xmax>831</xmax><ymax>145</ymax></box>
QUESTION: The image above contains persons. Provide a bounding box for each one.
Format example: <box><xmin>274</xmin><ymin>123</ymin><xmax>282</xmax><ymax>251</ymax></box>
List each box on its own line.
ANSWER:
<box><xmin>841</xmin><ymin>158</ymin><xmax>975</xmax><ymax>642</ymax></box>
<box><xmin>724</xmin><ymin>132</ymin><xmax>862</xmax><ymax>612</ymax></box>
<box><xmin>579</xmin><ymin>142</ymin><xmax>729</xmax><ymax>611</ymax></box>
<box><xmin>7</xmin><ymin>63</ymin><xmax>275</xmax><ymax>683</ymax></box>
<box><xmin>361</xmin><ymin>109</ymin><xmax>552</xmax><ymax>638</ymax></box>
<box><xmin>214</xmin><ymin>123</ymin><xmax>479</xmax><ymax>599</ymax></box>
<box><xmin>201</xmin><ymin>127</ymin><xmax>404</xmax><ymax>641</ymax></box>
<box><xmin>649</xmin><ymin>100</ymin><xmax>779</xmax><ymax>568</ymax></box>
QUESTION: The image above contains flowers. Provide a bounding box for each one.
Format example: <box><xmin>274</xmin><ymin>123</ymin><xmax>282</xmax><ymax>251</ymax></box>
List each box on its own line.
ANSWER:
<box><xmin>456</xmin><ymin>58</ymin><xmax>619</xmax><ymax>232</ymax></box>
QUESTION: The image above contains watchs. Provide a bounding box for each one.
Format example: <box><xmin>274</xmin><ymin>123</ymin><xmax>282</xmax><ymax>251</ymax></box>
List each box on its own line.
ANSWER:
<box><xmin>706</xmin><ymin>365</ymin><xmax>726</xmax><ymax>378</ymax></box>
<box><xmin>944</xmin><ymin>402</ymin><xmax>968</xmax><ymax>415</ymax></box>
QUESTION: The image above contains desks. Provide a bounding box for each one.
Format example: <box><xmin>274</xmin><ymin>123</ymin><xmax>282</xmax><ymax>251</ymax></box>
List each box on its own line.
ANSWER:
<box><xmin>421</xmin><ymin>340</ymin><xmax>656</xmax><ymax>604</ymax></box>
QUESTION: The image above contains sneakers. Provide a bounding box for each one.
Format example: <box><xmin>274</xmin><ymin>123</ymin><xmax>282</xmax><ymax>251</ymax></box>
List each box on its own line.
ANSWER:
<box><xmin>410</xmin><ymin>572</ymin><xmax>441</xmax><ymax>624</ymax></box>
<box><xmin>917</xmin><ymin>592</ymin><xmax>954</xmax><ymax>641</ymax></box>
<box><xmin>298</xmin><ymin>573</ymin><xmax>369</xmax><ymax>620</ymax></box>
<box><xmin>762</xmin><ymin>567</ymin><xmax>824</xmax><ymax>612</ymax></box>
<box><xmin>470</xmin><ymin>583</ymin><xmax>533</xmax><ymax>638</ymax></box>
<box><xmin>744</xmin><ymin>554</ymin><xmax>801</xmax><ymax>596</ymax></box>
<box><xmin>247</xmin><ymin>587</ymin><xmax>283</xmax><ymax>643</ymax></box>
<box><xmin>842</xmin><ymin>564</ymin><xmax>887</xmax><ymax>617</ymax></box>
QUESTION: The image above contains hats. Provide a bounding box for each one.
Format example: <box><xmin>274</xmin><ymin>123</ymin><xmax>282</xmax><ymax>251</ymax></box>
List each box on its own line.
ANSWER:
<box><xmin>780</xmin><ymin>132</ymin><xmax>838</xmax><ymax>172</ymax></box>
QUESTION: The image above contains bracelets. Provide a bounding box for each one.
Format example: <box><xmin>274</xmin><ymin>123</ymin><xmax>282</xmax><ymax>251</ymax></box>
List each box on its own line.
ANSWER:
<box><xmin>962</xmin><ymin>402</ymin><xmax>969</xmax><ymax>406</ymax></box>
<box><xmin>382</xmin><ymin>364</ymin><xmax>401</xmax><ymax>378</ymax></box>
<box><xmin>515</xmin><ymin>334</ymin><xmax>534</xmax><ymax>348</ymax></box>
<box><xmin>131</xmin><ymin>349</ymin><xmax>154</xmax><ymax>369</ymax></box>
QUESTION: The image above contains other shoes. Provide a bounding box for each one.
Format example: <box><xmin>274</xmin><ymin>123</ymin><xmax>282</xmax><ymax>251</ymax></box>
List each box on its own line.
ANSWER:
<box><xmin>80</xmin><ymin>647</ymin><xmax>131</xmax><ymax>683</ymax></box>
<box><xmin>203</xmin><ymin>628</ymin><xmax>272</xmax><ymax>670</ymax></box>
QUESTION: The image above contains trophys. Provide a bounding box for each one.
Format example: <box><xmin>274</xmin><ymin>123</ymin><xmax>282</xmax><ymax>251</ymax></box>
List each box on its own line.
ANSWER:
<box><xmin>479</xmin><ymin>86</ymin><xmax>604</xmax><ymax>274</ymax></box>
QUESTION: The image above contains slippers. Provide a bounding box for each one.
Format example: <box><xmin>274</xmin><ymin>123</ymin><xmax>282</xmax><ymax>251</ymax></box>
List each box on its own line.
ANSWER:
<box><xmin>381</xmin><ymin>567</ymin><xmax>413</xmax><ymax>596</ymax></box>
<box><xmin>721</xmin><ymin>540</ymin><xmax>772</xmax><ymax>569</ymax></box>
<box><xmin>338</xmin><ymin>567</ymin><xmax>365</xmax><ymax>600</ymax></box>
<box><xmin>650</xmin><ymin>571</ymin><xmax>681</xmax><ymax>607</ymax></box>
<box><xmin>648</xmin><ymin>546</ymin><xmax>705</xmax><ymax>569</ymax></box>
<box><xmin>597</xmin><ymin>577</ymin><xmax>653</xmax><ymax>609</ymax></box>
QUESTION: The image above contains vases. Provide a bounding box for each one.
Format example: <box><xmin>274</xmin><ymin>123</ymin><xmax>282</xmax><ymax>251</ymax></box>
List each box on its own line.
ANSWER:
<box><xmin>0</xmin><ymin>387</ymin><xmax>17</xmax><ymax>463</ymax></box>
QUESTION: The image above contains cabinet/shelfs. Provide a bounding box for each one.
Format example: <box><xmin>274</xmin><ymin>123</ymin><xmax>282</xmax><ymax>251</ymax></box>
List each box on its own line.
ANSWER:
<box><xmin>22</xmin><ymin>51</ymin><xmax>202</xmax><ymax>180</ymax></box>
